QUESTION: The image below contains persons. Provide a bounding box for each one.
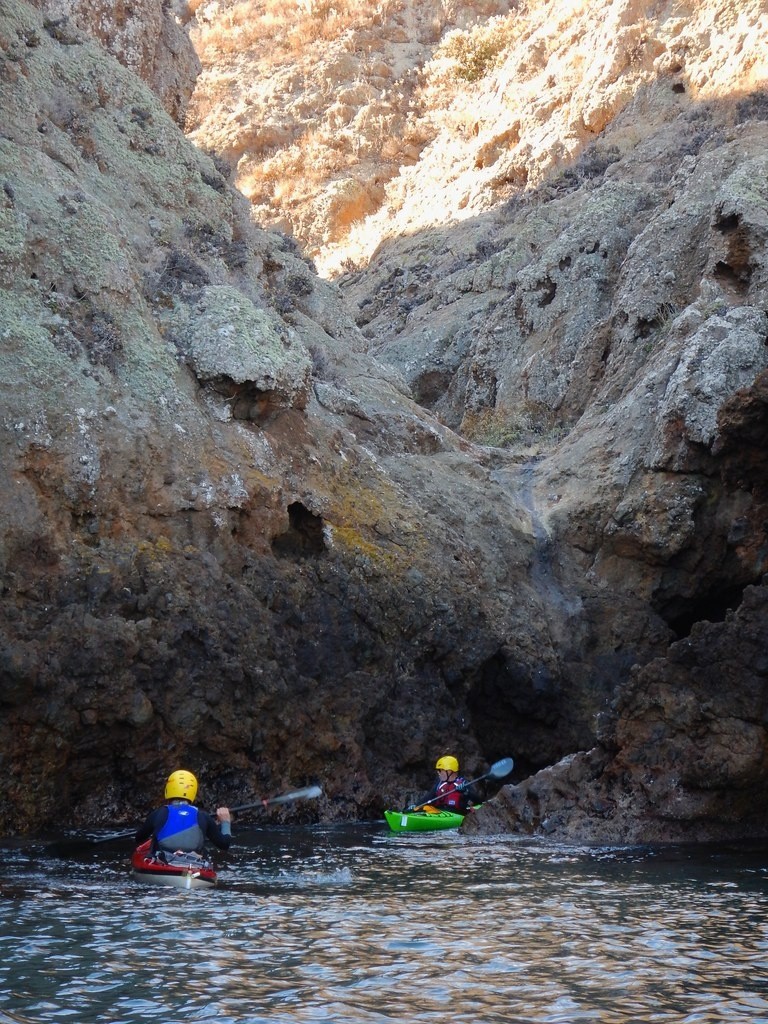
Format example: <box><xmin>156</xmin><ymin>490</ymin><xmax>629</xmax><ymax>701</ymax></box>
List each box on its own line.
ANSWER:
<box><xmin>134</xmin><ymin>770</ymin><xmax>232</xmax><ymax>864</ymax></box>
<box><xmin>408</xmin><ymin>755</ymin><xmax>483</xmax><ymax>816</ymax></box>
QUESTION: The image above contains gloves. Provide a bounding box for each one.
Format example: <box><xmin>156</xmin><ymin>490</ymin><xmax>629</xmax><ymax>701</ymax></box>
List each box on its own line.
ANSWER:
<box><xmin>407</xmin><ymin>805</ymin><xmax>416</xmax><ymax>811</ymax></box>
<box><xmin>456</xmin><ymin>780</ymin><xmax>468</xmax><ymax>793</ymax></box>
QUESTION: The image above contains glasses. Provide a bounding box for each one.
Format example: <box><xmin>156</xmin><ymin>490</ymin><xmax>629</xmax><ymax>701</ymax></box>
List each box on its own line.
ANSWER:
<box><xmin>437</xmin><ymin>769</ymin><xmax>444</xmax><ymax>772</ymax></box>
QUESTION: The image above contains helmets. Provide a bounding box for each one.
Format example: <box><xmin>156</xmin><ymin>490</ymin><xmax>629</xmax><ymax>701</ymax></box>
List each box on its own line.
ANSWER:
<box><xmin>164</xmin><ymin>769</ymin><xmax>198</xmax><ymax>805</ymax></box>
<box><xmin>436</xmin><ymin>756</ymin><xmax>459</xmax><ymax>772</ymax></box>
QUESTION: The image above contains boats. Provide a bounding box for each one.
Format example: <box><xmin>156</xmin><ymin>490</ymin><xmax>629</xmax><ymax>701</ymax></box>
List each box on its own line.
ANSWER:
<box><xmin>384</xmin><ymin>805</ymin><xmax>483</xmax><ymax>832</ymax></box>
<box><xmin>130</xmin><ymin>837</ymin><xmax>218</xmax><ymax>890</ymax></box>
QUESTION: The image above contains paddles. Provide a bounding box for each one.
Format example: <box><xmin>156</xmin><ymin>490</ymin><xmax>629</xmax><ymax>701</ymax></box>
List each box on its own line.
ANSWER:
<box><xmin>412</xmin><ymin>757</ymin><xmax>512</xmax><ymax>814</ymax></box>
<box><xmin>98</xmin><ymin>786</ymin><xmax>323</xmax><ymax>843</ymax></box>
